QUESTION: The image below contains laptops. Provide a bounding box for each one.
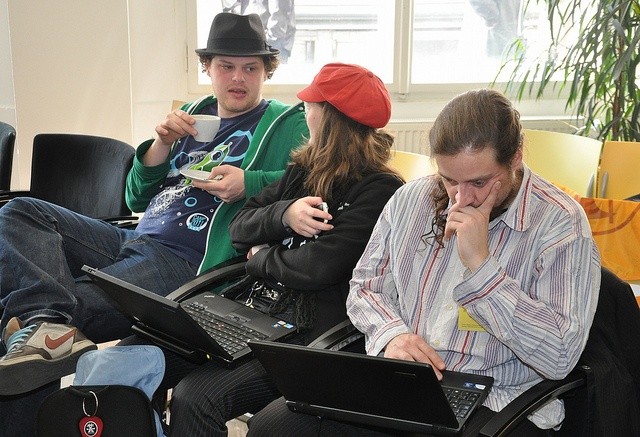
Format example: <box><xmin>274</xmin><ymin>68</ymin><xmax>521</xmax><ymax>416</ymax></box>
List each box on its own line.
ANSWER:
<box><xmin>246</xmin><ymin>340</ymin><xmax>494</xmax><ymax>437</ymax></box>
<box><xmin>80</xmin><ymin>264</ymin><xmax>298</xmax><ymax>371</ymax></box>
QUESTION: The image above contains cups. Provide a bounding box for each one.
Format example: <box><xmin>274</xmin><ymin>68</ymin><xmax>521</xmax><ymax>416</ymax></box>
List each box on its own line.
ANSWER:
<box><xmin>190</xmin><ymin>114</ymin><xmax>222</xmax><ymax>143</ymax></box>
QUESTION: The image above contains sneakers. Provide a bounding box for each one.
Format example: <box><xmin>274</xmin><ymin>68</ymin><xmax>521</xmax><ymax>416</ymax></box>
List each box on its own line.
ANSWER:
<box><xmin>0</xmin><ymin>316</ymin><xmax>98</xmax><ymax>395</ymax></box>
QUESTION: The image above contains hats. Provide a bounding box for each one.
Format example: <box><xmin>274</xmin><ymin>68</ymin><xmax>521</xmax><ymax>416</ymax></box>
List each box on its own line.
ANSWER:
<box><xmin>194</xmin><ymin>13</ymin><xmax>280</xmax><ymax>57</ymax></box>
<box><xmin>296</xmin><ymin>63</ymin><xmax>391</xmax><ymax>128</ymax></box>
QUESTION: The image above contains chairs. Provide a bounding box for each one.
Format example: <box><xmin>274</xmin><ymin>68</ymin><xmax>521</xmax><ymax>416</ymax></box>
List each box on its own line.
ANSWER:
<box><xmin>1</xmin><ymin>121</ymin><xmax>16</xmax><ymax>191</ymax></box>
<box><xmin>522</xmin><ymin>129</ymin><xmax>604</xmax><ymax>198</ymax></box>
<box><xmin>590</xmin><ymin>141</ymin><xmax>640</xmax><ymax>199</ymax></box>
<box><xmin>385</xmin><ymin>150</ymin><xmax>438</xmax><ymax>184</ymax></box>
<box><xmin>570</xmin><ymin>195</ymin><xmax>640</xmax><ymax>287</ymax></box>
<box><xmin>1</xmin><ymin>132</ymin><xmax>137</xmax><ymax>217</ymax></box>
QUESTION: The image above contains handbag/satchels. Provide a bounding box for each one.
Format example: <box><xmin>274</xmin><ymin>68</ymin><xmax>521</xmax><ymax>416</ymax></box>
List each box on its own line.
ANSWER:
<box><xmin>34</xmin><ymin>385</ymin><xmax>158</xmax><ymax>437</ymax></box>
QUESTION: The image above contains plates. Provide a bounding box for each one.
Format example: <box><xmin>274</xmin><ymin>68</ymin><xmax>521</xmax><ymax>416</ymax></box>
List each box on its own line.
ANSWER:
<box><xmin>180</xmin><ymin>170</ymin><xmax>224</xmax><ymax>183</ymax></box>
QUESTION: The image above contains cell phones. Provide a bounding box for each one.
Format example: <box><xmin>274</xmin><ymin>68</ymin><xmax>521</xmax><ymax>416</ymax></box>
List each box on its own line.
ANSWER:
<box><xmin>311</xmin><ymin>203</ymin><xmax>329</xmax><ymax>223</ymax></box>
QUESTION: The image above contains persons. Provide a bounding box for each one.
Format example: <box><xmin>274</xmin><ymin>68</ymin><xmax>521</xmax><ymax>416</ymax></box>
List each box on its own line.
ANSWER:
<box><xmin>246</xmin><ymin>87</ymin><xmax>601</xmax><ymax>435</ymax></box>
<box><xmin>220</xmin><ymin>0</ymin><xmax>296</xmax><ymax>63</ymax></box>
<box><xmin>0</xmin><ymin>11</ymin><xmax>312</xmax><ymax>436</ymax></box>
<box><xmin>470</xmin><ymin>0</ymin><xmax>526</xmax><ymax>81</ymax></box>
<box><xmin>114</xmin><ymin>63</ymin><xmax>406</xmax><ymax>437</ymax></box>
